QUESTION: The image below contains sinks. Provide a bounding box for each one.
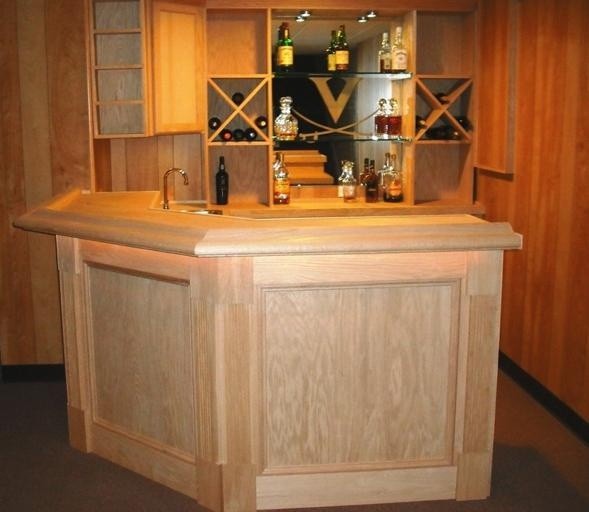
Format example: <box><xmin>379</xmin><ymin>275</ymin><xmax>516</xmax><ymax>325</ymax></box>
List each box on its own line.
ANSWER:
<box><xmin>181</xmin><ymin>209</ymin><xmax>224</xmax><ymax>215</ymax></box>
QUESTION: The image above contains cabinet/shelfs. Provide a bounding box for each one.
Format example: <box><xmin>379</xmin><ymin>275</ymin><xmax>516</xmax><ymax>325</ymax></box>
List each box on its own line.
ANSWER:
<box><xmin>88</xmin><ymin>0</ymin><xmax>479</xmax><ymax>211</ymax></box>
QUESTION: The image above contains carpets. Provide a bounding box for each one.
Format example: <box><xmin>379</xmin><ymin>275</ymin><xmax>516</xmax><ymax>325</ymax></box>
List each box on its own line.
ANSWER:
<box><xmin>0</xmin><ymin>381</ymin><xmax>587</xmax><ymax>511</ymax></box>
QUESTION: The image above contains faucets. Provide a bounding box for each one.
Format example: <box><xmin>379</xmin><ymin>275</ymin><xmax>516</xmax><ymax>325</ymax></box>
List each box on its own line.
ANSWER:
<box><xmin>162</xmin><ymin>167</ymin><xmax>188</xmax><ymax>209</ymax></box>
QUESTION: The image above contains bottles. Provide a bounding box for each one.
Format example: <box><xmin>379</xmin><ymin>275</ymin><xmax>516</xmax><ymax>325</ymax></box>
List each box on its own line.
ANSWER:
<box><xmin>335</xmin><ymin>24</ymin><xmax>350</xmax><ymax>71</ymax></box>
<box><xmin>388</xmin><ymin>96</ymin><xmax>402</xmax><ymax>140</ymax></box>
<box><xmin>416</xmin><ymin>114</ymin><xmax>467</xmax><ymax>140</ymax></box>
<box><xmin>275</xmin><ymin>21</ymin><xmax>294</xmax><ymax>72</ymax></box>
<box><xmin>326</xmin><ymin>30</ymin><xmax>336</xmax><ymax>72</ymax></box>
<box><xmin>375</xmin><ymin>97</ymin><xmax>389</xmax><ymax>138</ymax></box>
<box><xmin>274</xmin><ymin>96</ymin><xmax>299</xmax><ymax>140</ymax></box>
<box><xmin>377</xmin><ymin>31</ymin><xmax>392</xmax><ymax>74</ymax></box>
<box><xmin>337</xmin><ymin>150</ymin><xmax>390</xmax><ymax>186</ymax></box>
<box><xmin>364</xmin><ymin>159</ymin><xmax>379</xmax><ymax>203</ymax></box>
<box><xmin>383</xmin><ymin>153</ymin><xmax>403</xmax><ymax>202</ymax></box>
<box><xmin>209</xmin><ymin>115</ymin><xmax>267</xmax><ymax>141</ymax></box>
<box><xmin>273</xmin><ymin>153</ymin><xmax>290</xmax><ymax>205</ymax></box>
<box><xmin>342</xmin><ymin>161</ymin><xmax>357</xmax><ymax>202</ymax></box>
<box><xmin>231</xmin><ymin>92</ymin><xmax>244</xmax><ymax>105</ymax></box>
<box><xmin>392</xmin><ymin>25</ymin><xmax>407</xmax><ymax>72</ymax></box>
<box><xmin>434</xmin><ymin>91</ymin><xmax>449</xmax><ymax>103</ymax></box>
<box><xmin>215</xmin><ymin>155</ymin><xmax>229</xmax><ymax>205</ymax></box>
